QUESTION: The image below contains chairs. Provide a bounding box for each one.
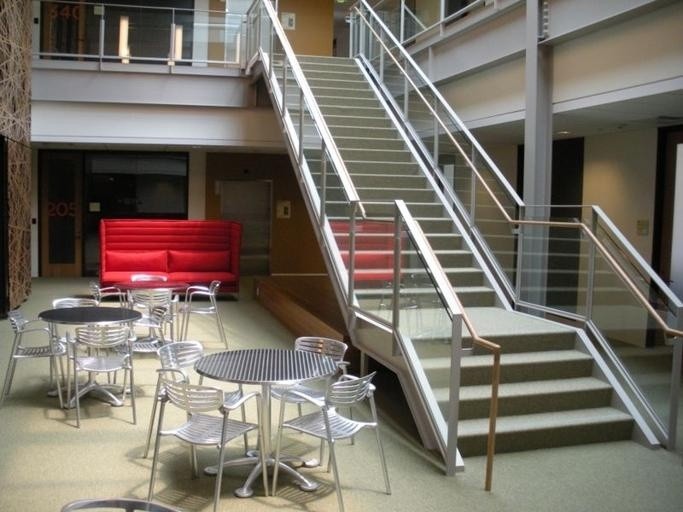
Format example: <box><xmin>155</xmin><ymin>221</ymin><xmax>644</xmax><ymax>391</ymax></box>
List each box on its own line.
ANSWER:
<box><xmin>3</xmin><ymin>310</ymin><xmax>66</xmax><ymax>407</ymax></box>
<box><xmin>0</xmin><ymin>269</ymin><xmax>180</xmax><ymax>382</ymax></box>
<box><xmin>271</xmin><ymin>371</ymin><xmax>390</xmax><ymax>510</ymax></box>
<box><xmin>293</xmin><ymin>336</ymin><xmax>354</xmax><ymax>447</ymax></box>
<box><xmin>61</xmin><ymin>497</ymin><xmax>190</xmax><ymax>512</ymax></box>
<box><xmin>144</xmin><ymin>341</ymin><xmax>205</xmax><ymax>458</ymax></box>
<box><xmin>180</xmin><ymin>279</ymin><xmax>229</xmax><ymax>349</ymax></box>
<box><xmin>68</xmin><ymin>325</ymin><xmax>137</xmax><ymax>427</ymax></box>
<box><xmin>149</xmin><ymin>378</ymin><xmax>269</xmax><ymax>510</ymax></box>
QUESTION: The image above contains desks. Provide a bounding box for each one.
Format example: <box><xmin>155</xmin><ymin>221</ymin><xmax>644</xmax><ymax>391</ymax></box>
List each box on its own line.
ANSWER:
<box><xmin>195</xmin><ymin>348</ymin><xmax>338</xmax><ymax>498</ymax></box>
<box><xmin>38</xmin><ymin>307</ymin><xmax>143</xmax><ymax>408</ymax></box>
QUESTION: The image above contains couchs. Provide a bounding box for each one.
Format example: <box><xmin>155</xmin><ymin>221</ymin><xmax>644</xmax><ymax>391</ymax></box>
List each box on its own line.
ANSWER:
<box><xmin>97</xmin><ymin>218</ymin><xmax>243</xmax><ymax>300</ymax></box>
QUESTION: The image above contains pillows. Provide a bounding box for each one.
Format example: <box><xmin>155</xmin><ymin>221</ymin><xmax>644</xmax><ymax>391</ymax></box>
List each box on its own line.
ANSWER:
<box><xmin>105</xmin><ymin>249</ymin><xmax>169</xmax><ymax>273</ymax></box>
<box><xmin>166</xmin><ymin>248</ymin><xmax>230</xmax><ymax>271</ymax></box>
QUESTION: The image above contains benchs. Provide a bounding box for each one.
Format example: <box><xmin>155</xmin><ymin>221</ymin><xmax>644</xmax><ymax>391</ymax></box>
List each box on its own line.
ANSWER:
<box><xmin>252</xmin><ymin>254</ymin><xmax>357</xmax><ymax>361</ymax></box>
